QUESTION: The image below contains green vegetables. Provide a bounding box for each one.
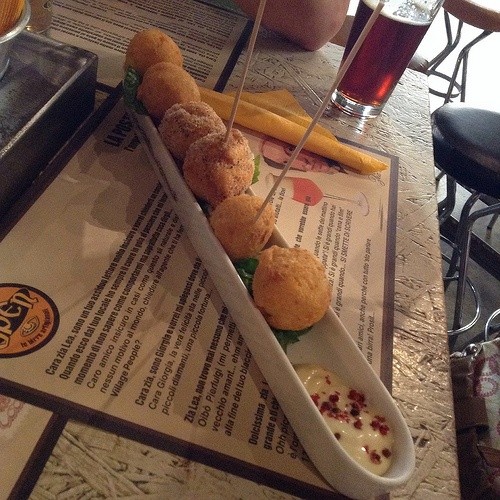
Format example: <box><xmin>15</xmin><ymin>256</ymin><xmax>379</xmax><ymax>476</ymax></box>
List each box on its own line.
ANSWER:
<box><xmin>125</xmin><ymin>66</ymin><xmax>312</xmax><ymax>347</ymax></box>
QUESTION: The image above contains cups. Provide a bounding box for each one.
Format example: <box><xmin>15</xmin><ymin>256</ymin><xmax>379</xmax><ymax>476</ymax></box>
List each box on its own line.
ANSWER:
<box><xmin>330</xmin><ymin>1</ymin><xmax>444</xmax><ymax>119</ymax></box>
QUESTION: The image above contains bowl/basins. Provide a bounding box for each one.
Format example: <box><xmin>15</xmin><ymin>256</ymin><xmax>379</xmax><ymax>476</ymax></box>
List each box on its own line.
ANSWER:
<box><xmin>0</xmin><ymin>0</ymin><xmax>31</xmax><ymax>79</ymax></box>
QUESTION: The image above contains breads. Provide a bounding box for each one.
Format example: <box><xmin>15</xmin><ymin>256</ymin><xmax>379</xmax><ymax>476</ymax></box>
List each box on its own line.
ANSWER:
<box><xmin>123</xmin><ymin>29</ymin><xmax>183</xmax><ymax>76</ymax></box>
<box><xmin>136</xmin><ymin>62</ymin><xmax>202</xmax><ymax>120</ymax></box>
<box><xmin>209</xmin><ymin>194</ymin><xmax>275</xmax><ymax>259</ymax></box>
<box><xmin>182</xmin><ymin>128</ymin><xmax>255</xmax><ymax>208</ymax></box>
<box><xmin>158</xmin><ymin>100</ymin><xmax>226</xmax><ymax>161</ymax></box>
<box><xmin>252</xmin><ymin>245</ymin><xmax>332</xmax><ymax>330</ymax></box>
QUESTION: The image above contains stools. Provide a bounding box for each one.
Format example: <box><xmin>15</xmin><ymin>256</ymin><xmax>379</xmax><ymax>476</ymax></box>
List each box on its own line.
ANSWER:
<box><xmin>428</xmin><ymin>101</ymin><xmax>500</xmax><ymax>350</ymax></box>
<box><xmin>421</xmin><ymin>0</ymin><xmax>500</xmax><ymax>106</ymax></box>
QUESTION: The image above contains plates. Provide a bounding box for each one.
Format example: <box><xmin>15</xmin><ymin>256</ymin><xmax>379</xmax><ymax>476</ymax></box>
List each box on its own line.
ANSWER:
<box><xmin>120</xmin><ymin>53</ymin><xmax>417</xmax><ymax>500</ymax></box>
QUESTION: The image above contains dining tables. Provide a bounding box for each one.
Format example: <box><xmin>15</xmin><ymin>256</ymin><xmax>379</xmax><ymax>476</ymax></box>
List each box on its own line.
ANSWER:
<box><xmin>23</xmin><ymin>26</ymin><xmax>464</xmax><ymax>500</ymax></box>
